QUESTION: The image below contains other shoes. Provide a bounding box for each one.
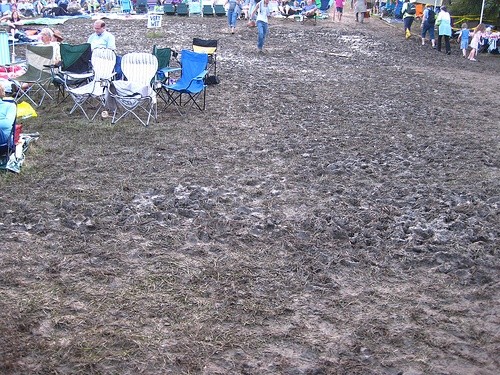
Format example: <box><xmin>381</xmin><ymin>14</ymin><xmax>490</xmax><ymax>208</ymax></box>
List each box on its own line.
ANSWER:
<box><xmin>470</xmin><ymin>58</ymin><xmax>476</xmax><ymax>61</ymax></box>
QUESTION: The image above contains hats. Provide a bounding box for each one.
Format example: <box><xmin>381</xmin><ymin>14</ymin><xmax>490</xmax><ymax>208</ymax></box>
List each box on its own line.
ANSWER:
<box><xmin>425</xmin><ymin>3</ymin><xmax>431</xmax><ymax>7</ymax></box>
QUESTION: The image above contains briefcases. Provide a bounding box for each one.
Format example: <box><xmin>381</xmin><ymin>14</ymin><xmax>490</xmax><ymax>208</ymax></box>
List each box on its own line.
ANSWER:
<box><xmin>361</xmin><ymin>11</ymin><xmax>370</xmax><ymax>18</ymax></box>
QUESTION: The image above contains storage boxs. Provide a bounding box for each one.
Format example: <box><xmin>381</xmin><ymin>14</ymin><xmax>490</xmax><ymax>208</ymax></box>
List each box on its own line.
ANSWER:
<box><xmin>15</xmin><ymin>124</ymin><xmax>23</xmax><ymax>143</ymax></box>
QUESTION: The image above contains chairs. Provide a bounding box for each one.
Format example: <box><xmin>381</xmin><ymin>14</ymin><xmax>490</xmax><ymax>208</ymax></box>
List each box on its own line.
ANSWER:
<box><xmin>176</xmin><ymin>3</ymin><xmax>189</xmax><ymax>16</ymax></box>
<box><xmin>136</xmin><ymin>0</ymin><xmax>148</xmax><ymax>13</ymax></box>
<box><xmin>193</xmin><ymin>38</ymin><xmax>219</xmax><ymax>85</ymax></box>
<box><xmin>111</xmin><ymin>55</ymin><xmax>126</xmax><ymax>81</ymax></box>
<box><xmin>214</xmin><ymin>5</ymin><xmax>225</xmax><ymax>16</ymax></box>
<box><xmin>152</xmin><ymin>45</ymin><xmax>177</xmax><ymax>104</ymax></box>
<box><xmin>164</xmin><ymin>4</ymin><xmax>176</xmax><ymax>15</ymax></box>
<box><xmin>8</xmin><ymin>43</ymin><xmax>56</xmax><ymax>106</ymax></box>
<box><xmin>0</xmin><ymin>29</ymin><xmax>11</xmax><ymax>65</ymax></box>
<box><xmin>0</xmin><ymin>100</ymin><xmax>17</xmax><ymax>171</ymax></box>
<box><xmin>203</xmin><ymin>5</ymin><xmax>214</xmax><ymax>17</ymax></box>
<box><xmin>189</xmin><ymin>2</ymin><xmax>202</xmax><ymax>17</ymax></box>
<box><xmin>100</xmin><ymin>52</ymin><xmax>159</xmax><ymax>127</ymax></box>
<box><xmin>158</xmin><ymin>50</ymin><xmax>209</xmax><ymax>111</ymax></box>
<box><xmin>63</xmin><ymin>47</ymin><xmax>117</xmax><ymax>123</ymax></box>
<box><xmin>44</xmin><ymin>43</ymin><xmax>94</xmax><ymax>108</ymax></box>
<box><xmin>120</xmin><ymin>0</ymin><xmax>132</xmax><ymax>14</ymax></box>
<box><xmin>280</xmin><ymin>2</ymin><xmax>317</xmax><ymax>25</ymax></box>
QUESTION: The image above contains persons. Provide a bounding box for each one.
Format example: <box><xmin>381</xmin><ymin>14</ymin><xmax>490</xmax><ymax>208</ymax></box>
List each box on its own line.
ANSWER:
<box><xmin>251</xmin><ymin>0</ymin><xmax>270</xmax><ymax>51</ymax></box>
<box><xmin>435</xmin><ymin>6</ymin><xmax>452</xmax><ymax>55</ymax></box>
<box><xmin>420</xmin><ymin>4</ymin><xmax>436</xmax><ymax>49</ymax></box>
<box><xmin>0</xmin><ymin>81</ymin><xmax>17</xmax><ymax>145</ymax></box>
<box><xmin>457</xmin><ymin>22</ymin><xmax>470</xmax><ymax>57</ymax></box>
<box><xmin>334</xmin><ymin>0</ymin><xmax>346</xmax><ymax>20</ymax></box>
<box><xmin>468</xmin><ymin>24</ymin><xmax>485</xmax><ymax>62</ymax></box>
<box><xmin>36</xmin><ymin>27</ymin><xmax>61</xmax><ymax>64</ymax></box>
<box><xmin>157</xmin><ymin>0</ymin><xmax>258</xmax><ymax>34</ymax></box>
<box><xmin>402</xmin><ymin>0</ymin><xmax>416</xmax><ymax>39</ymax></box>
<box><xmin>0</xmin><ymin>0</ymin><xmax>149</xmax><ymax>45</ymax></box>
<box><xmin>272</xmin><ymin>0</ymin><xmax>318</xmax><ymax>16</ymax></box>
<box><xmin>354</xmin><ymin>0</ymin><xmax>386</xmax><ymax>24</ymax></box>
<box><xmin>87</xmin><ymin>20</ymin><xmax>116</xmax><ymax>60</ymax></box>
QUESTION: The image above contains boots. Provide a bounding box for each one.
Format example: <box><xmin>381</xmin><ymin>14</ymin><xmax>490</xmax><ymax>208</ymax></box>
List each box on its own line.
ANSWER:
<box><xmin>422</xmin><ymin>38</ymin><xmax>425</xmax><ymax>45</ymax></box>
<box><xmin>432</xmin><ymin>39</ymin><xmax>437</xmax><ymax>48</ymax></box>
<box><xmin>405</xmin><ymin>28</ymin><xmax>411</xmax><ymax>39</ymax></box>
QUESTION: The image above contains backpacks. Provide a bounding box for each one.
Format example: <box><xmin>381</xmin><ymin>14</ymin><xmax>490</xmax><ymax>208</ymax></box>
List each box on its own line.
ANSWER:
<box><xmin>428</xmin><ymin>9</ymin><xmax>436</xmax><ymax>23</ymax></box>
<box><xmin>405</xmin><ymin>2</ymin><xmax>416</xmax><ymax>14</ymax></box>
